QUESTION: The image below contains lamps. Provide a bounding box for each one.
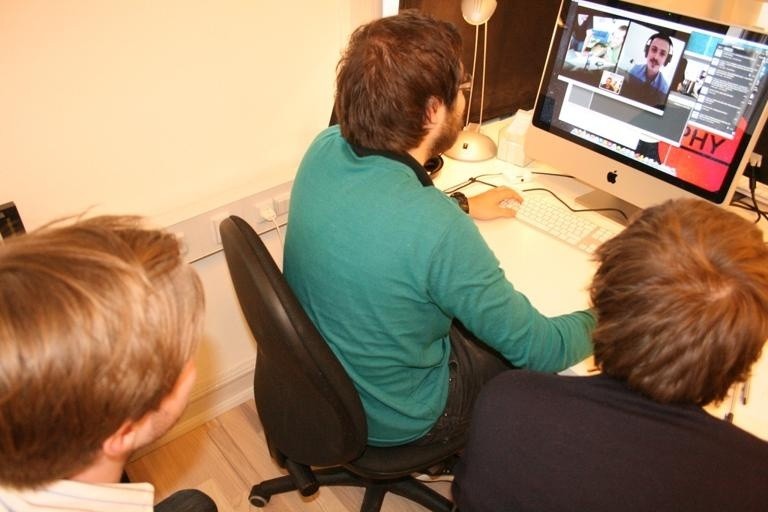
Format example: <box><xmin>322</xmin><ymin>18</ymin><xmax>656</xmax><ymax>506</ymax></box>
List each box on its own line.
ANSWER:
<box><xmin>443</xmin><ymin>0</ymin><xmax>496</xmax><ymax>161</ymax></box>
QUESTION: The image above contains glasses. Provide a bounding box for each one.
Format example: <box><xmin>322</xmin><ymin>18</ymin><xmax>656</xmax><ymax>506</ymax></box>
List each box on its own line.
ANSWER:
<box><xmin>457</xmin><ymin>71</ymin><xmax>473</xmax><ymax>92</ymax></box>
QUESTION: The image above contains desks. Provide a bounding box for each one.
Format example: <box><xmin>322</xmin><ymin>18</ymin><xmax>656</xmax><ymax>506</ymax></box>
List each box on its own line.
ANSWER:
<box><xmin>421</xmin><ymin>106</ymin><xmax>768</xmax><ymax>443</ymax></box>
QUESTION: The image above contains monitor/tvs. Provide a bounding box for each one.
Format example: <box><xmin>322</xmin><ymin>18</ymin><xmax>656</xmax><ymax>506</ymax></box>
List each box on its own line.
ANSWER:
<box><xmin>528</xmin><ymin>0</ymin><xmax>768</xmax><ymax>205</ymax></box>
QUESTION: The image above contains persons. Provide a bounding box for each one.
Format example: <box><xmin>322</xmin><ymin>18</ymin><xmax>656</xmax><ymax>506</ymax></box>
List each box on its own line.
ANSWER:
<box><xmin>602</xmin><ymin>78</ymin><xmax>614</xmax><ymax>92</ymax></box>
<box><xmin>570</xmin><ymin>13</ymin><xmax>626</xmax><ymax>67</ymax></box>
<box><xmin>451</xmin><ymin>197</ymin><xmax>768</xmax><ymax>512</ymax></box>
<box><xmin>284</xmin><ymin>8</ymin><xmax>599</xmax><ymax>448</ymax></box>
<box><xmin>630</xmin><ymin>33</ymin><xmax>673</xmax><ymax>93</ymax></box>
<box><xmin>0</xmin><ymin>205</ymin><xmax>216</xmax><ymax>512</ymax></box>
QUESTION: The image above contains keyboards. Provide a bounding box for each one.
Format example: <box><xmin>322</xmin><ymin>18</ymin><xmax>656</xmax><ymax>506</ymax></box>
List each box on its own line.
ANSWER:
<box><xmin>497</xmin><ymin>194</ymin><xmax>619</xmax><ymax>255</ymax></box>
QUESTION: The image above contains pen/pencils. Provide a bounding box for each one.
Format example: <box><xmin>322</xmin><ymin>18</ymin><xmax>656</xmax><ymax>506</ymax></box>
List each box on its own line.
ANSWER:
<box><xmin>725</xmin><ymin>368</ymin><xmax>751</xmax><ymax>424</ymax></box>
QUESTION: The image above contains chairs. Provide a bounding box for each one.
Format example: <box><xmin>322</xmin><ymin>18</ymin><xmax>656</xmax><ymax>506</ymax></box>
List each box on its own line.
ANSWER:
<box><xmin>221</xmin><ymin>216</ymin><xmax>471</xmax><ymax>512</ymax></box>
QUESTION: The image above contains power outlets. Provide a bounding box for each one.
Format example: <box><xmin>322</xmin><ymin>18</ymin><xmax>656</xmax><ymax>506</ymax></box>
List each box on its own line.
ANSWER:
<box><xmin>255</xmin><ymin>199</ymin><xmax>275</xmax><ymax>224</ymax></box>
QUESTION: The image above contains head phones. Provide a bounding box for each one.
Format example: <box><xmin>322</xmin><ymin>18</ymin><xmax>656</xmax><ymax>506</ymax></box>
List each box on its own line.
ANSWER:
<box><xmin>644</xmin><ymin>34</ymin><xmax>674</xmax><ymax>66</ymax></box>
